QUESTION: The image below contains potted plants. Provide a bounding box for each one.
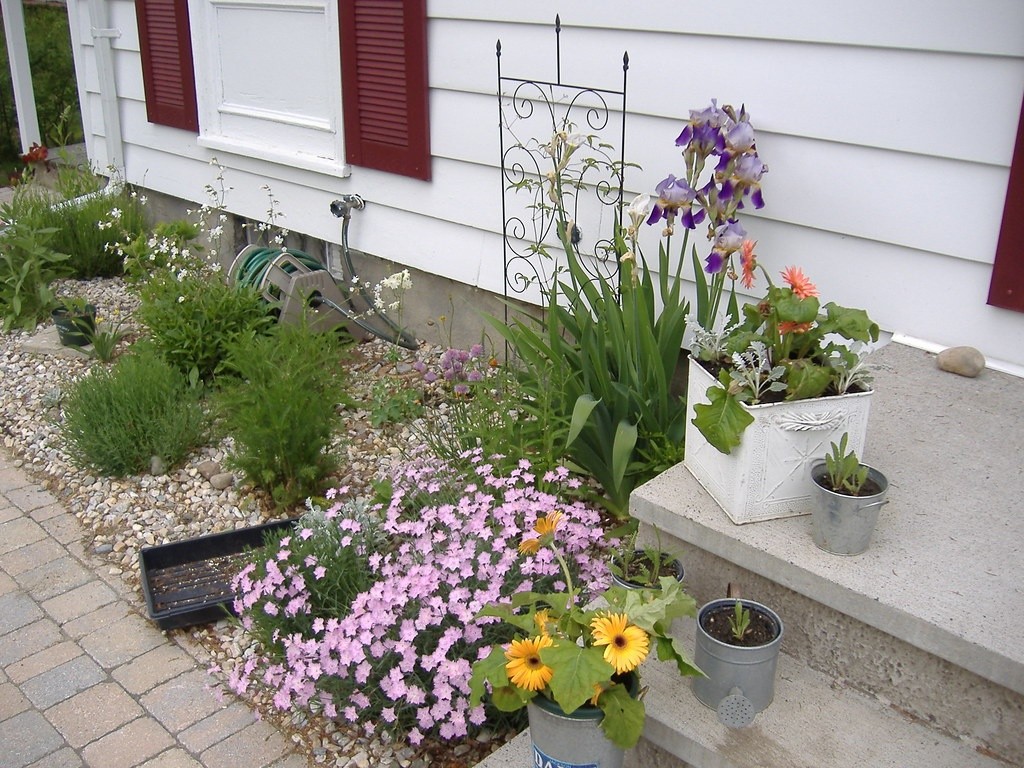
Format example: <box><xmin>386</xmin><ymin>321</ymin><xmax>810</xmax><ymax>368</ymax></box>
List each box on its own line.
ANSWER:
<box><xmin>605</xmin><ymin>522</ymin><xmax>686</xmax><ymax>590</ymax></box>
<box><xmin>51</xmin><ymin>295</ymin><xmax>97</xmax><ymax>346</ymax></box>
<box><xmin>691</xmin><ymin>597</ymin><xmax>784</xmax><ymax>713</ymax></box>
<box><xmin>807</xmin><ymin>430</ymin><xmax>890</xmax><ymax>555</ymax></box>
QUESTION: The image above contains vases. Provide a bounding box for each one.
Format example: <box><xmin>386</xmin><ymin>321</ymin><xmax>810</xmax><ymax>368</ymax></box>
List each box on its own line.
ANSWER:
<box><xmin>683</xmin><ymin>353</ymin><xmax>875</xmax><ymax>525</ymax></box>
<box><xmin>527</xmin><ymin>647</ymin><xmax>639</xmax><ymax>768</ymax></box>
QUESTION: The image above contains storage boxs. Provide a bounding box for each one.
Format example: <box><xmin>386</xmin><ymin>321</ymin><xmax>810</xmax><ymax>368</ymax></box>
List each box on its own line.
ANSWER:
<box><xmin>139</xmin><ymin>518</ymin><xmax>301</xmax><ymax>630</ymax></box>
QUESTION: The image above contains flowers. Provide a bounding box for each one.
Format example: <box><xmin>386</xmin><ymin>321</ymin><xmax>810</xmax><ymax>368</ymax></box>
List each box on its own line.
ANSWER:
<box><xmin>467</xmin><ymin>511</ymin><xmax>712</xmax><ymax>749</ymax></box>
<box><xmin>644</xmin><ymin>97</ymin><xmax>880</xmax><ymax>456</ymax></box>
